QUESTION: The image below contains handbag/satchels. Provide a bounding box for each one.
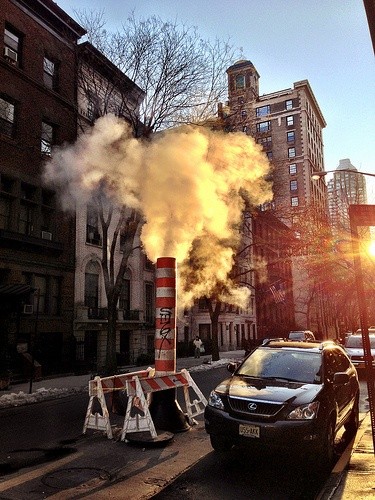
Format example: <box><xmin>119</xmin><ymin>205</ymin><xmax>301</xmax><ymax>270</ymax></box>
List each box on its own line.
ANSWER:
<box><xmin>200</xmin><ymin>347</ymin><xmax>205</xmax><ymax>352</ymax></box>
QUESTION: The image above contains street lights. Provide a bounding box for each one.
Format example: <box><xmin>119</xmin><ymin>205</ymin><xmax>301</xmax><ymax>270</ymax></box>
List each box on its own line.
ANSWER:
<box><xmin>310</xmin><ymin>169</ymin><xmax>375</xmax><ymax>181</ymax></box>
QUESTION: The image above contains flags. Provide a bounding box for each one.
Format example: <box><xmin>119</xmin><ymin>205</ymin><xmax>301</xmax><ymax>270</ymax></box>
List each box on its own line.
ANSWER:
<box><xmin>269</xmin><ymin>279</ymin><xmax>286</xmax><ymax>304</ymax></box>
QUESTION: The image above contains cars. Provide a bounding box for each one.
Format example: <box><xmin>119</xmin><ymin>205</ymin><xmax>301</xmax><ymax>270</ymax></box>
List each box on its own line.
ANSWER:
<box><xmin>287</xmin><ymin>330</ymin><xmax>315</xmax><ymax>339</ymax></box>
<box><xmin>339</xmin><ymin>326</ymin><xmax>375</xmax><ymax>374</ymax></box>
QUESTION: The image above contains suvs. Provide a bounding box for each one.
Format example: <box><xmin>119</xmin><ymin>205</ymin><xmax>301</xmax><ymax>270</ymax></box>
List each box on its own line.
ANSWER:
<box><xmin>204</xmin><ymin>339</ymin><xmax>361</xmax><ymax>473</ymax></box>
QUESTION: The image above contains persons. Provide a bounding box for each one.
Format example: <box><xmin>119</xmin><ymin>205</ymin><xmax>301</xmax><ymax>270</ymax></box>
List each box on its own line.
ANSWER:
<box><xmin>193</xmin><ymin>336</ymin><xmax>203</xmax><ymax>358</ymax></box>
